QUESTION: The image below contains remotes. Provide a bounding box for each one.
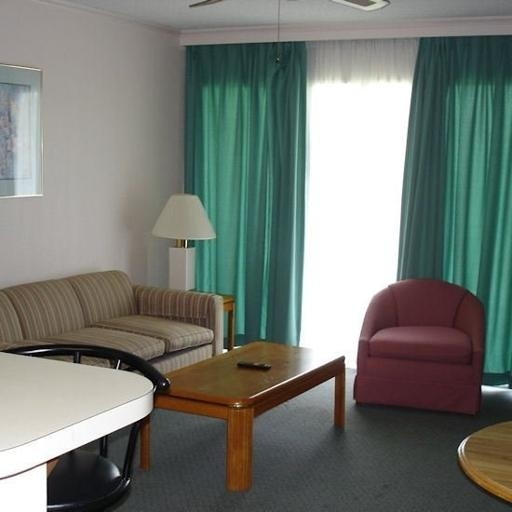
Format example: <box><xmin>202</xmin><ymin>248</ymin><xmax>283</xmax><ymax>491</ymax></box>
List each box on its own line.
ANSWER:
<box><xmin>237</xmin><ymin>360</ymin><xmax>272</xmax><ymax>370</ymax></box>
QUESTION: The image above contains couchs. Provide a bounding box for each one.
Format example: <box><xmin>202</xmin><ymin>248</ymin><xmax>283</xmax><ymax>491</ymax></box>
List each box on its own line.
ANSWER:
<box><xmin>0</xmin><ymin>270</ymin><xmax>223</xmax><ymax>377</ymax></box>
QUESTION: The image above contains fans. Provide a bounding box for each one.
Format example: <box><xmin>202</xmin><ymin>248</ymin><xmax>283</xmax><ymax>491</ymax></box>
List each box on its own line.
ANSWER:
<box><xmin>188</xmin><ymin>0</ymin><xmax>391</xmax><ymax>64</ymax></box>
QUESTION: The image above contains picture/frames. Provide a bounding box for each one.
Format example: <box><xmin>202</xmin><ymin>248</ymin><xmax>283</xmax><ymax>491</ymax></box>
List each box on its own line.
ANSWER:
<box><xmin>0</xmin><ymin>62</ymin><xmax>44</xmax><ymax>200</ymax></box>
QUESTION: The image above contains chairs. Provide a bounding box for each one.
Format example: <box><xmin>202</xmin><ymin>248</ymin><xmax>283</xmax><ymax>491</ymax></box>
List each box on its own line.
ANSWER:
<box><xmin>352</xmin><ymin>280</ymin><xmax>486</xmax><ymax>416</ymax></box>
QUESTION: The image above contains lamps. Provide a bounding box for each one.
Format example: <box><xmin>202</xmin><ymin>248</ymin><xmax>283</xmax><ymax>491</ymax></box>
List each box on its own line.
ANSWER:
<box><xmin>151</xmin><ymin>193</ymin><xmax>217</xmax><ymax>291</ymax></box>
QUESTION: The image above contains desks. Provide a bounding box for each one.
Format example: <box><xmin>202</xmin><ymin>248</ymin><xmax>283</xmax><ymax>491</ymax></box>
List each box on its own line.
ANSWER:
<box><xmin>456</xmin><ymin>420</ymin><xmax>512</xmax><ymax>502</ymax></box>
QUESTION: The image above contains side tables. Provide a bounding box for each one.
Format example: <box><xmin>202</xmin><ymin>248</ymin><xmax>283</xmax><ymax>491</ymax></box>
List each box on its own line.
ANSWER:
<box><xmin>223</xmin><ymin>297</ymin><xmax>236</xmax><ymax>351</ymax></box>
